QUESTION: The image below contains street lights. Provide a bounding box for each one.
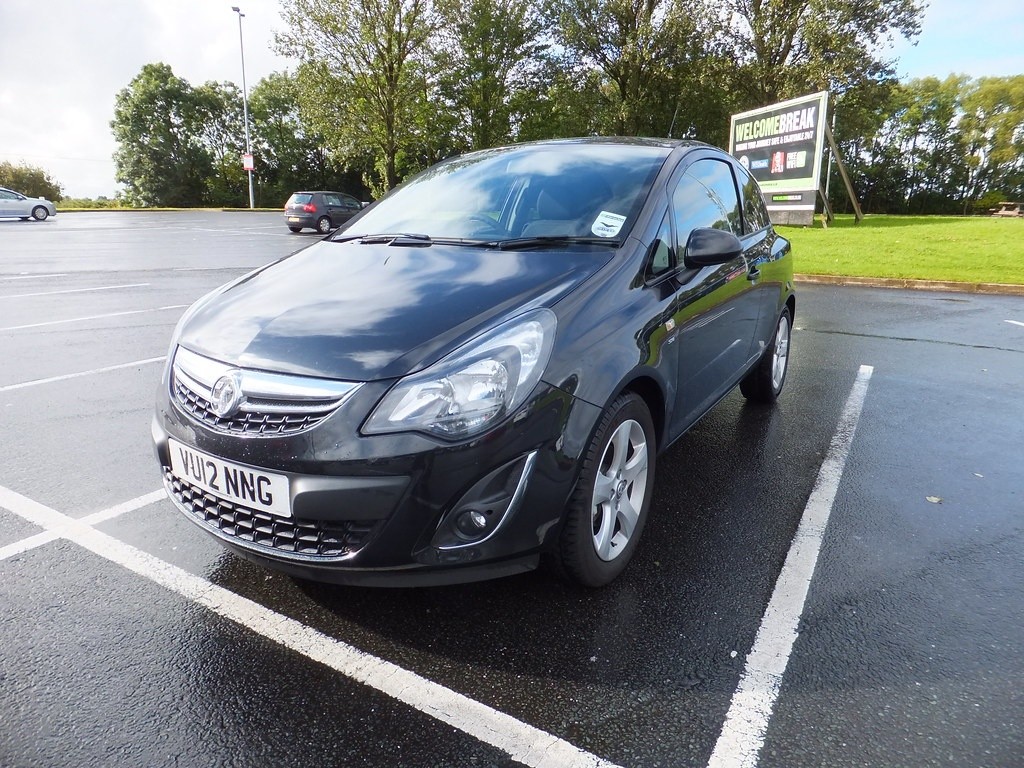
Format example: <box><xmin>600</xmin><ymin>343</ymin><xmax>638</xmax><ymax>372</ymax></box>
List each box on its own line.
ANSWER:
<box><xmin>231</xmin><ymin>5</ymin><xmax>259</xmax><ymax>209</ymax></box>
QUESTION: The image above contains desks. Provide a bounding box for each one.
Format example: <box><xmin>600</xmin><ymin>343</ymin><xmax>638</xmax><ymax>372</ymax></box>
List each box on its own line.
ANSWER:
<box><xmin>993</xmin><ymin>202</ymin><xmax>1024</xmax><ymax>217</ymax></box>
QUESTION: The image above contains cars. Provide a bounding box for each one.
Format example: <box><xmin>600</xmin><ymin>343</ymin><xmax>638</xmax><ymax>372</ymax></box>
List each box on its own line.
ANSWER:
<box><xmin>155</xmin><ymin>133</ymin><xmax>794</xmax><ymax>594</ymax></box>
<box><xmin>0</xmin><ymin>188</ymin><xmax>57</xmax><ymax>221</ymax></box>
<box><xmin>283</xmin><ymin>190</ymin><xmax>367</xmax><ymax>233</ymax></box>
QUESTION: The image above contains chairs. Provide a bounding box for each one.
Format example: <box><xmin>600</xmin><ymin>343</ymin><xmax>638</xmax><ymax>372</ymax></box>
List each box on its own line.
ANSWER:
<box><xmin>520</xmin><ymin>186</ymin><xmax>580</xmax><ymax>240</ymax></box>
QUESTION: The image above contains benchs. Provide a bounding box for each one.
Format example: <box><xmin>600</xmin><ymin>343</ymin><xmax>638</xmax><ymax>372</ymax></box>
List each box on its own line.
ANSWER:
<box><xmin>990</xmin><ymin>208</ymin><xmax>1001</xmax><ymax>212</ymax></box>
